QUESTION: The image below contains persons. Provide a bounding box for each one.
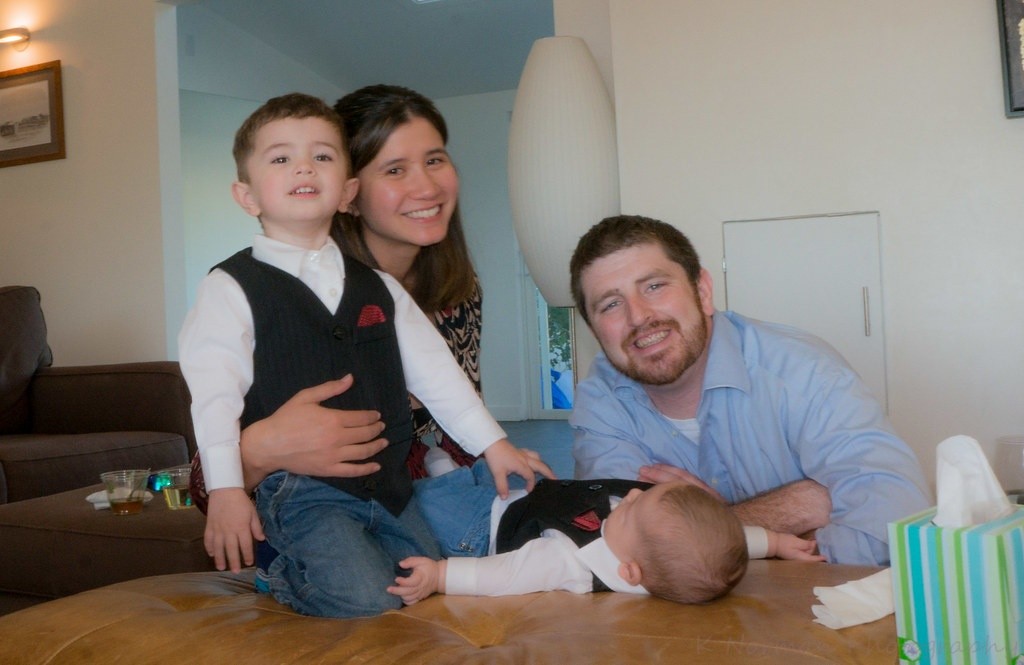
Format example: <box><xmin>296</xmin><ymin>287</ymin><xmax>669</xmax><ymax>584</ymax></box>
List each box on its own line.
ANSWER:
<box><xmin>173</xmin><ymin>93</ymin><xmax>560</xmax><ymax>621</ymax></box>
<box><xmin>388</xmin><ymin>446</ymin><xmax>829</xmax><ymax>607</ymax></box>
<box><xmin>563</xmin><ymin>214</ymin><xmax>934</xmax><ymax>575</ymax></box>
<box><xmin>181</xmin><ymin>83</ymin><xmax>485</xmax><ymax>521</ymax></box>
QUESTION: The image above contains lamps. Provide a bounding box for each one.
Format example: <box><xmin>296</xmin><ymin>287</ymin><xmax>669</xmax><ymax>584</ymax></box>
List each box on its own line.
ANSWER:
<box><xmin>0</xmin><ymin>28</ymin><xmax>30</xmax><ymax>51</ymax></box>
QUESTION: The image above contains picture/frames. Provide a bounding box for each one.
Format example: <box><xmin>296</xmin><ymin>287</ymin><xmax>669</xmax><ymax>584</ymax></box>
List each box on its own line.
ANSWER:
<box><xmin>996</xmin><ymin>0</ymin><xmax>1024</xmax><ymax>118</ymax></box>
<box><xmin>0</xmin><ymin>60</ymin><xmax>66</xmax><ymax>167</ymax></box>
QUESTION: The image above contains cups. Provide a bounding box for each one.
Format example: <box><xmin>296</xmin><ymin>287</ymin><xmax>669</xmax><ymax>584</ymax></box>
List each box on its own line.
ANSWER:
<box><xmin>157</xmin><ymin>466</ymin><xmax>196</xmax><ymax>510</ymax></box>
<box><xmin>994</xmin><ymin>434</ymin><xmax>1024</xmax><ymax>490</ymax></box>
<box><xmin>101</xmin><ymin>469</ymin><xmax>153</xmax><ymax>515</ymax></box>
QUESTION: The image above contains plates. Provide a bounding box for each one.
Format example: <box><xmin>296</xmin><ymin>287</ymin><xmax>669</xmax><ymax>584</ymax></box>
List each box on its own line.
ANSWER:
<box><xmin>85</xmin><ymin>487</ymin><xmax>154</xmax><ymax>510</ymax></box>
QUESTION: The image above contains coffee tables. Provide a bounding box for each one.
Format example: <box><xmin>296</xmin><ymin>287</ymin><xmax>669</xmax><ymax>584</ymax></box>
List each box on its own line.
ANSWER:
<box><xmin>0</xmin><ymin>464</ymin><xmax>256</xmax><ymax>620</ymax></box>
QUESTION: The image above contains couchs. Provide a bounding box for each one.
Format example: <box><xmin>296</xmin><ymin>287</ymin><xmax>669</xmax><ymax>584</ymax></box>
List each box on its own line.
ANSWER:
<box><xmin>0</xmin><ymin>286</ymin><xmax>197</xmax><ymax>505</ymax></box>
<box><xmin>0</xmin><ymin>556</ymin><xmax>899</xmax><ymax>665</ymax></box>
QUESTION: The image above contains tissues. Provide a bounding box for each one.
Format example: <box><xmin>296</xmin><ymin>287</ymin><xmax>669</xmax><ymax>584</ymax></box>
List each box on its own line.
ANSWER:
<box><xmin>889</xmin><ymin>433</ymin><xmax>1024</xmax><ymax>664</ymax></box>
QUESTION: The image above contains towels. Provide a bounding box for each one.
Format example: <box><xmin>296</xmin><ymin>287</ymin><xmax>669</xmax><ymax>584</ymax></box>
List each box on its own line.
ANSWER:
<box><xmin>811</xmin><ymin>565</ymin><xmax>894</xmax><ymax>631</ymax></box>
